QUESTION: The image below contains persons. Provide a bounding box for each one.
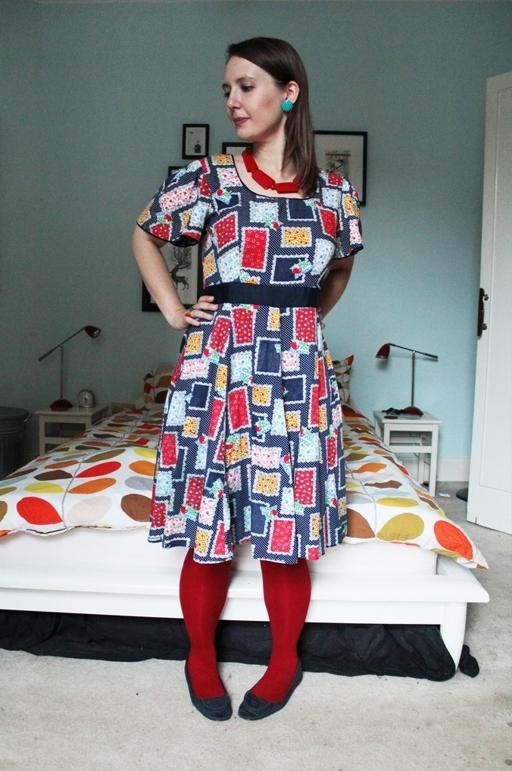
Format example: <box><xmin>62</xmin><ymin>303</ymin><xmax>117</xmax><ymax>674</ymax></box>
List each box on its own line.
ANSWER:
<box><xmin>130</xmin><ymin>36</ymin><xmax>365</xmax><ymax>722</ymax></box>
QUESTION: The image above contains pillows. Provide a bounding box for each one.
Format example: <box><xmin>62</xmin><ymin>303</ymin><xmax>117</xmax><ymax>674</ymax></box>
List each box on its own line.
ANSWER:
<box><xmin>139</xmin><ymin>359</ymin><xmax>178</xmax><ymax>410</ymax></box>
<box><xmin>332</xmin><ymin>354</ymin><xmax>355</xmax><ymax>407</ymax></box>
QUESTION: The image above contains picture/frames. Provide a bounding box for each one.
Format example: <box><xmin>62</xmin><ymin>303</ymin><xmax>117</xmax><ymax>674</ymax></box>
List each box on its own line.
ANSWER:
<box><xmin>313</xmin><ymin>130</ymin><xmax>366</xmax><ymax>207</ymax></box>
<box><xmin>182</xmin><ymin>123</ymin><xmax>209</xmax><ymax>159</ymax></box>
<box><xmin>222</xmin><ymin>142</ymin><xmax>252</xmax><ymax>155</ymax></box>
<box><xmin>142</xmin><ymin>243</ymin><xmax>201</xmax><ymax>312</ymax></box>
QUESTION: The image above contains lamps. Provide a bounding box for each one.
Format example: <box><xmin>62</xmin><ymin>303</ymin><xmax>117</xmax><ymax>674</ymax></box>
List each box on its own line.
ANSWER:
<box><xmin>376</xmin><ymin>342</ymin><xmax>438</xmax><ymax>419</ymax></box>
<box><xmin>39</xmin><ymin>325</ymin><xmax>103</xmax><ymax>412</ymax></box>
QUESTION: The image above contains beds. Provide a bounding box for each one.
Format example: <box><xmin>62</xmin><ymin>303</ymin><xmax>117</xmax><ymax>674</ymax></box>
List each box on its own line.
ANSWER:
<box><xmin>0</xmin><ymin>407</ymin><xmax>492</xmax><ymax>673</ymax></box>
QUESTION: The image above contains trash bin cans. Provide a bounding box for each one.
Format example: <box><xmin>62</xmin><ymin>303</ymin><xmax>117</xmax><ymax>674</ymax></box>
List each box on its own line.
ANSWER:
<box><xmin>0</xmin><ymin>407</ymin><xmax>32</xmax><ymax>476</ymax></box>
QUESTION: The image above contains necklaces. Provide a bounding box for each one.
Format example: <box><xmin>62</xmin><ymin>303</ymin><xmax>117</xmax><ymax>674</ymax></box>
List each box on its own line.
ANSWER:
<box><xmin>241</xmin><ymin>150</ymin><xmax>303</xmax><ymax>193</ymax></box>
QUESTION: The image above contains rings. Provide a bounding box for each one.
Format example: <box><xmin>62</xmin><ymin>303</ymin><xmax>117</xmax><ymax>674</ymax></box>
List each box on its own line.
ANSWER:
<box><xmin>185</xmin><ymin>306</ymin><xmax>195</xmax><ymax>318</ymax></box>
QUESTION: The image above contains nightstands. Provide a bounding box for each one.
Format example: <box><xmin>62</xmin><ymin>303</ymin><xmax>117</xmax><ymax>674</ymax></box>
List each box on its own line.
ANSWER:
<box><xmin>374</xmin><ymin>409</ymin><xmax>441</xmax><ymax>498</ymax></box>
<box><xmin>34</xmin><ymin>404</ymin><xmax>113</xmax><ymax>457</ymax></box>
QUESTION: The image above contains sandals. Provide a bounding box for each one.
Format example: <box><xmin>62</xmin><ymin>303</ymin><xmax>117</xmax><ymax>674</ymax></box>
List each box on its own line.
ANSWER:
<box><xmin>184</xmin><ymin>656</ymin><xmax>303</xmax><ymax>720</ymax></box>
